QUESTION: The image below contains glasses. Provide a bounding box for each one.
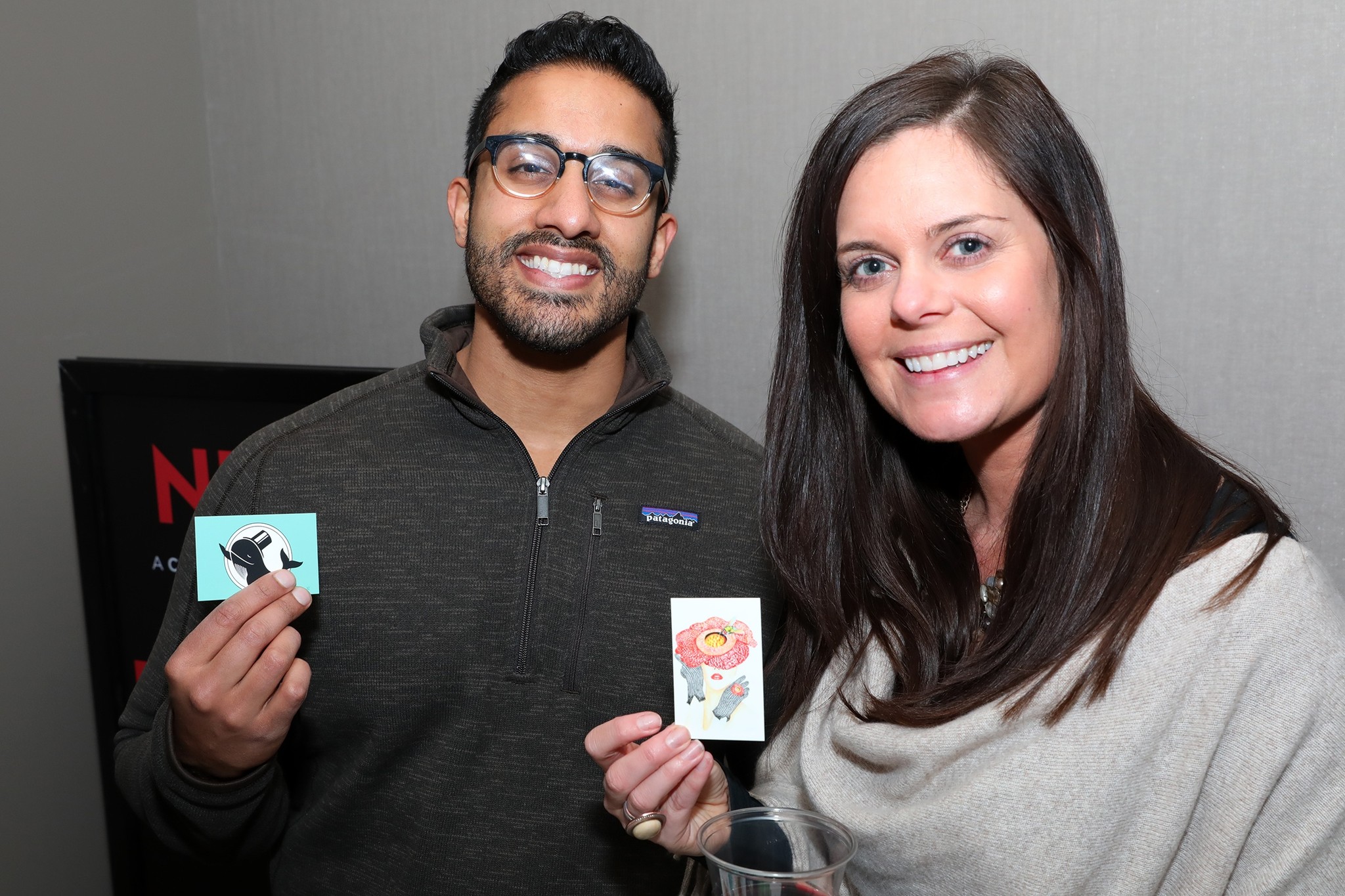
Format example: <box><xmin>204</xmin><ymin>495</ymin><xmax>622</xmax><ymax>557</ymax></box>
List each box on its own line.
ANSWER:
<box><xmin>467</xmin><ymin>134</ymin><xmax>670</xmax><ymax>215</ymax></box>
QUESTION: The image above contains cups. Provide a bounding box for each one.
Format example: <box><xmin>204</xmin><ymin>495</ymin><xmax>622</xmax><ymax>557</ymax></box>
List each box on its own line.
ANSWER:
<box><xmin>696</xmin><ymin>806</ymin><xmax>858</xmax><ymax>896</ymax></box>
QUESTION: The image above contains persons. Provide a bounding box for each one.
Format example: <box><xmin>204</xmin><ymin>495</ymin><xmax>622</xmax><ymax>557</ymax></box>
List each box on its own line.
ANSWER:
<box><xmin>113</xmin><ymin>10</ymin><xmax>779</xmax><ymax>896</ymax></box>
<box><xmin>582</xmin><ymin>38</ymin><xmax>1344</xmax><ymax>896</ymax></box>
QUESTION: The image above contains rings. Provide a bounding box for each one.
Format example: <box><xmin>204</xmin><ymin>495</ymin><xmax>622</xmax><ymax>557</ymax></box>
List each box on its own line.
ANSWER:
<box><xmin>623</xmin><ymin>801</ymin><xmax>666</xmax><ymax>840</ymax></box>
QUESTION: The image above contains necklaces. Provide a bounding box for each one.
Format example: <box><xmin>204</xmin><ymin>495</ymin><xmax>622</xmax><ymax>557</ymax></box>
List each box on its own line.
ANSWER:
<box><xmin>957</xmin><ymin>489</ymin><xmax>1004</xmax><ymax>632</ymax></box>
